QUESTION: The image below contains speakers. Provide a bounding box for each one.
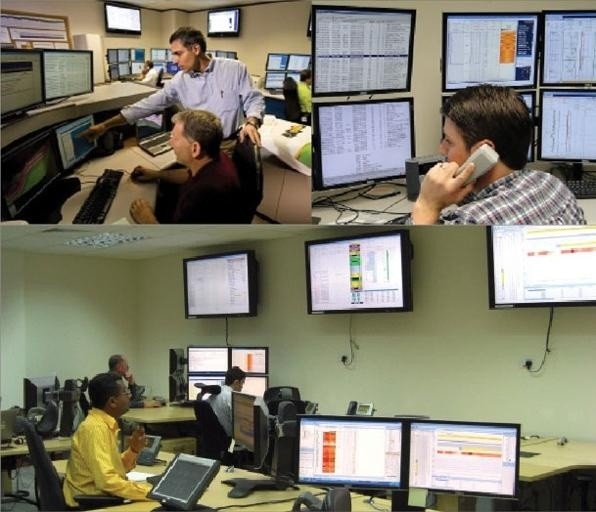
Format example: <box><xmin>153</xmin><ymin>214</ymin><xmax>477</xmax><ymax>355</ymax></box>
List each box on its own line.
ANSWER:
<box><xmin>406</xmin><ymin>159</ymin><xmax>420</xmax><ymax>201</ymax></box>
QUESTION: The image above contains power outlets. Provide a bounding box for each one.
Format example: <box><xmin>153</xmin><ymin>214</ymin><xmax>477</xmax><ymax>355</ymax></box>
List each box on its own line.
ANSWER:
<box><xmin>337</xmin><ymin>351</ymin><xmax>351</xmax><ymax>370</ymax></box>
<box><xmin>520</xmin><ymin>356</ymin><xmax>535</xmax><ymax>371</ymax></box>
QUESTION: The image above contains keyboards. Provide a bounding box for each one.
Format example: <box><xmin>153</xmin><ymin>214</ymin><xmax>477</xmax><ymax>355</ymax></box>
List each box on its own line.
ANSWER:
<box><xmin>562</xmin><ymin>179</ymin><xmax>596</xmax><ymax>198</ymax></box>
<box><xmin>138</xmin><ymin>131</ymin><xmax>173</xmax><ymax>156</ymax></box>
<box><xmin>72</xmin><ymin>169</ymin><xmax>124</xmax><ymax>224</ymax></box>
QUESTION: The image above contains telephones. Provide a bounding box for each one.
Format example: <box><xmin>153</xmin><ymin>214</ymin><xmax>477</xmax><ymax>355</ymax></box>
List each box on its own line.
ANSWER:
<box><xmin>453</xmin><ymin>143</ymin><xmax>500</xmax><ymax>187</ymax></box>
<box><xmin>137</xmin><ymin>435</ymin><xmax>167</xmax><ymax>465</ymax></box>
<box><xmin>347</xmin><ymin>401</ymin><xmax>375</xmax><ymax>416</ymax></box>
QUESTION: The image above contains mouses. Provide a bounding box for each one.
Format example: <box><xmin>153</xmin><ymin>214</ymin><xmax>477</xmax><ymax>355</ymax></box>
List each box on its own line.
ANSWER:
<box><xmin>131</xmin><ymin>172</ymin><xmax>143</xmax><ymax>178</ymax></box>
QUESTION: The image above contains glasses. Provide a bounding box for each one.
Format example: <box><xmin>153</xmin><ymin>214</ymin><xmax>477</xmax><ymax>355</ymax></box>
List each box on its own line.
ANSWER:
<box><xmin>120</xmin><ymin>389</ymin><xmax>131</xmax><ymax>395</ymax></box>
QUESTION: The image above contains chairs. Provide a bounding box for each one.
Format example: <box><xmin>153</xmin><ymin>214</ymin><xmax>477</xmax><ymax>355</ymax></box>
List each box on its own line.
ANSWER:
<box><xmin>283</xmin><ymin>76</ymin><xmax>312</xmax><ymax>124</ymax></box>
<box><xmin>155</xmin><ymin>67</ymin><xmax>166</xmax><ymax>88</ymax></box>
<box><xmin>15</xmin><ymin>415</ymin><xmax>128</xmax><ymax>511</ymax></box>
<box><xmin>192</xmin><ymin>401</ymin><xmax>252</xmax><ymax>469</ymax></box>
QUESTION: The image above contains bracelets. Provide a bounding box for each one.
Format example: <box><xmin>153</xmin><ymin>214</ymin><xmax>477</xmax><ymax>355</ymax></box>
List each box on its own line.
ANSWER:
<box><xmin>245</xmin><ymin>121</ymin><xmax>258</xmax><ymax>130</ymax></box>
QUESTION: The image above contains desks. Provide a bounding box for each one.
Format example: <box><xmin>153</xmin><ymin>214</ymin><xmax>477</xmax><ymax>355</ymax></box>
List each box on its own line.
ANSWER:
<box><xmin>1</xmin><ymin>451</ymin><xmax>440</xmax><ymax>512</ymax></box>
<box><xmin>58</xmin><ymin>132</ymin><xmax>177</xmax><ymax>224</ymax></box>
<box><xmin>435</xmin><ymin>436</ymin><xmax>596</xmax><ymax>512</ymax></box>
<box><xmin>116</xmin><ymin>404</ymin><xmax>196</xmax><ymax>454</ymax></box>
<box><xmin>0</xmin><ymin>437</ymin><xmax>74</xmax><ymax>471</ymax></box>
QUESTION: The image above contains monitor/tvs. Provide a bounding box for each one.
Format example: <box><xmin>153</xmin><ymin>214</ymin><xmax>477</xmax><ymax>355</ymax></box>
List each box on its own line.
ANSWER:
<box><xmin>104</xmin><ymin>3</ymin><xmax>142</xmax><ymax>35</ymax></box>
<box><xmin>285</xmin><ymin>72</ymin><xmax>301</xmax><ymax>81</ymax></box>
<box><xmin>150</xmin><ymin>48</ymin><xmax>166</xmax><ymax>62</ymax></box>
<box><xmin>166</xmin><ymin>62</ymin><xmax>180</xmax><ymax>75</ymax></box>
<box><xmin>264</xmin><ymin>72</ymin><xmax>286</xmax><ymax>89</ymax></box>
<box><xmin>221</xmin><ymin>387</ymin><xmax>318</xmax><ymax>499</ymax></box>
<box><xmin>107</xmin><ymin>64</ymin><xmax>119</xmax><ymax>81</ymax></box>
<box><xmin>296</xmin><ymin>414</ymin><xmax>406</xmax><ymax>491</ymax></box>
<box><xmin>239</xmin><ymin>376</ymin><xmax>268</xmax><ymax>406</ymax></box>
<box><xmin>265</xmin><ymin>53</ymin><xmax>289</xmax><ymax>71</ymax></box>
<box><xmin>105</xmin><ymin>49</ymin><xmax>118</xmax><ymax>64</ymax></box>
<box><xmin>0</xmin><ymin>48</ymin><xmax>44</xmax><ymax>124</ymax></box>
<box><xmin>117</xmin><ymin>63</ymin><xmax>130</xmax><ymax>77</ymax></box>
<box><xmin>207</xmin><ymin>8</ymin><xmax>240</xmax><ymax>36</ymax></box>
<box><xmin>313</xmin><ymin>5</ymin><xmax>416</xmax><ymax>96</ymax></box>
<box><xmin>441</xmin><ymin>12</ymin><xmax>540</xmax><ymax>90</ymax></box>
<box><xmin>441</xmin><ymin>91</ymin><xmax>535</xmax><ymax>163</ymax></box>
<box><xmin>151</xmin><ymin>62</ymin><xmax>167</xmax><ymax>74</ymax></box>
<box><xmin>540</xmin><ymin>9</ymin><xmax>596</xmax><ymax>87</ymax></box>
<box><xmin>23</xmin><ymin>376</ymin><xmax>59</xmax><ymax>415</ymax></box>
<box><xmin>312</xmin><ymin>96</ymin><xmax>416</xmax><ymax>192</ymax></box>
<box><xmin>230</xmin><ymin>346</ymin><xmax>268</xmax><ymax>376</ymax></box>
<box><xmin>183</xmin><ymin>249</ymin><xmax>259</xmax><ymax>318</ymax></box>
<box><xmin>169</xmin><ymin>377</ymin><xmax>185</xmax><ymax>402</ymax></box>
<box><xmin>304</xmin><ymin>230</ymin><xmax>413</xmax><ymax>314</ymax></box>
<box><xmin>486</xmin><ymin>225</ymin><xmax>596</xmax><ymax>310</ymax></box>
<box><xmin>166</xmin><ymin>49</ymin><xmax>173</xmax><ymax>62</ymax></box>
<box><xmin>187</xmin><ymin>375</ymin><xmax>226</xmax><ymax>402</ymax></box>
<box><xmin>130</xmin><ymin>48</ymin><xmax>145</xmax><ymax>63</ymax></box>
<box><xmin>130</xmin><ymin>61</ymin><xmax>146</xmax><ymax>75</ymax></box>
<box><xmin>1</xmin><ymin>129</ymin><xmax>62</xmax><ymax>221</ymax></box>
<box><xmin>117</xmin><ymin>49</ymin><xmax>129</xmax><ymax>63</ymax></box>
<box><xmin>188</xmin><ymin>345</ymin><xmax>230</xmax><ymax>377</ymax></box>
<box><xmin>536</xmin><ymin>89</ymin><xmax>596</xmax><ymax>181</ymax></box>
<box><xmin>205</xmin><ymin>50</ymin><xmax>216</xmax><ymax>60</ymax></box>
<box><xmin>406</xmin><ymin>420</ymin><xmax>520</xmax><ymax>501</ymax></box>
<box><xmin>286</xmin><ymin>54</ymin><xmax>311</xmax><ymax>72</ymax></box>
<box><xmin>42</xmin><ymin>49</ymin><xmax>93</xmax><ymax>100</ymax></box>
<box><xmin>215</xmin><ymin>50</ymin><xmax>236</xmax><ymax>60</ymax></box>
<box><xmin>146</xmin><ymin>452</ymin><xmax>221</xmax><ymax>509</ymax></box>
<box><xmin>51</xmin><ymin>114</ymin><xmax>98</xmax><ymax>173</ymax></box>
<box><xmin>169</xmin><ymin>348</ymin><xmax>184</xmax><ymax>377</ymax></box>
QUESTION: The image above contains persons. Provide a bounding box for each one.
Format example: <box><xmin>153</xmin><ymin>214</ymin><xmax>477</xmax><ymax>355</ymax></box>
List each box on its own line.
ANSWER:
<box><xmin>297</xmin><ymin>70</ymin><xmax>312</xmax><ymax>115</ymax></box>
<box><xmin>63</xmin><ymin>372</ymin><xmax>160</xmax><ymax>507</ymax></box>
<box><xmin>134</xmin><ymin>60</ymin><xmax>158</xmax><ymax>88</ymax></box>
<box><xmin>129</xmin><ymin>110</ymin><xmax>243</xmax><ymax>224</ymax></box>
<box><xmin>78</xmin><ymin>25</ymin><xmax>266</xmax><ymax>148</ymax></box>
<box><xmin>108</xmin><ymin>355</ymin><xmax>162</xmax><ymax>409</ymax></box>
<box><xmin>206</xmin><ymin>366</ymin><xmax>246</xmax><ymax>439</ymax></box>
<box><xmin>409</xmin><ymin>83</ymin><xmax>589</xmax><ymax>225</ymax></box>
